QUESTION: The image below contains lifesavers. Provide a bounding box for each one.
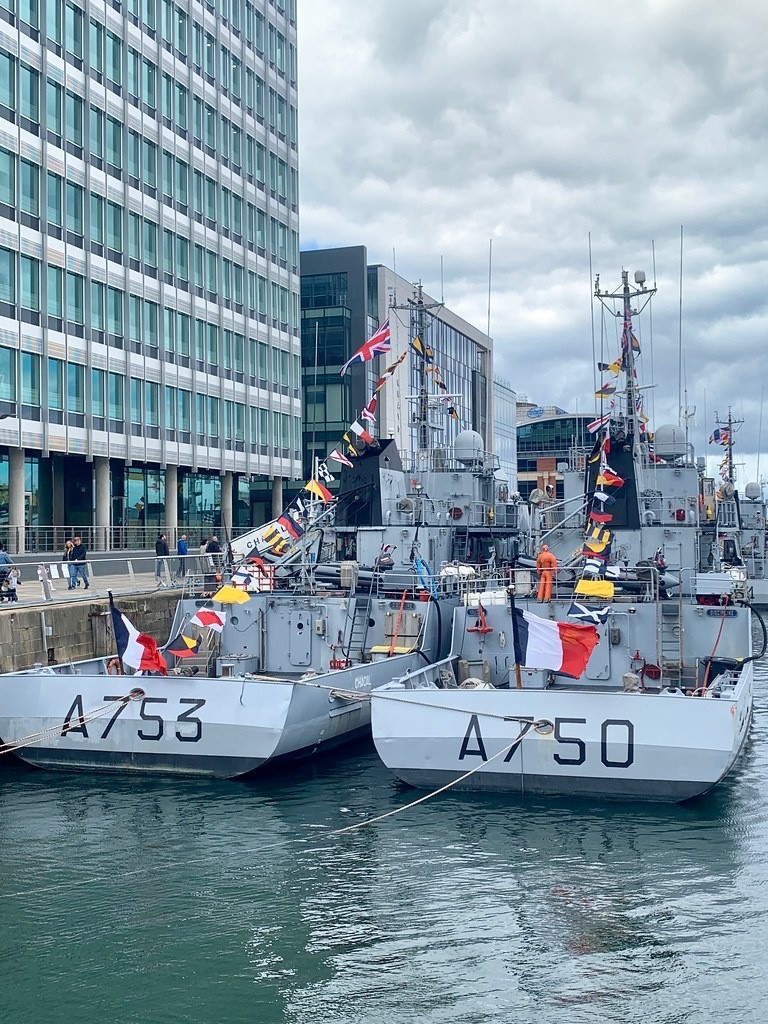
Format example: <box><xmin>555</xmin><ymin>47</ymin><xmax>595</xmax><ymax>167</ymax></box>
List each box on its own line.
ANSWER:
<box><xmin>699</xmin><ymin>494</ymin><xmax>703</xmax><ymax>512</ymax></box>
<box><xmin>107</xmin><ymin>659</ymin><xmax>122</xmax><ymax>675</ymax></box>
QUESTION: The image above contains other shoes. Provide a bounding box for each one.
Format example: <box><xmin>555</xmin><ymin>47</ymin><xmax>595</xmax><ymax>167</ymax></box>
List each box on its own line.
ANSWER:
<box><xmin>68</xmin><ymin>586</ymin><xmax>76</xmax><ymax>590</ymax></box>
<box><xmin>544</xmin><ymin>600</ymin><xmax>551</xmax><ymax>603</ymax></box>
<box><xmin>76</xmin><ymin>581</ymin><xmax>80</xmax><ymax>586</ymax></box>
<box><xmin>537</xmin><ymin>599</ymin><xmax>543</xmax><ymax>603</ymax></box>
<box><xmin>84</xmin><ymin>586</ymin><xmax>89</xmax><ymax>589</ymax></box>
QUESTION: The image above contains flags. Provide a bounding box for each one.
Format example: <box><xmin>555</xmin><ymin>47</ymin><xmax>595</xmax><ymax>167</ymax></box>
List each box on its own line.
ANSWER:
<box><xmin>340</xmin><ymin>317</ymin><xmax>391</xmax><ymax>378</ymax></box>
<box><xmin>109</xmin><ymin>593</ymin><xmax>169</xmax><ymax>676</ymax></box>
<box><xmin>563</xmin><ymin>309</ymin><xmax>737</xmax><ymax>627</ymax></box>
<box><xmin>162</xmin><ymin>335</ymin><xmax>462</xmax><ymax>676</ymax></box>
<box><xmin>512</xmin><ymin>607</ymin><xmax>600</xmax><ymax>681</ymax></box>
<box><xmin>135</xmin><ymin>497</ymin><xmax>145</xmax><ymax>511</ymax></box>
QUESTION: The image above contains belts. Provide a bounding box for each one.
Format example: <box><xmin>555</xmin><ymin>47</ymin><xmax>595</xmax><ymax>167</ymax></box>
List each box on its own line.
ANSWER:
<box><xmin>543</xmin><ymin>569</ymin><xmax>551</xmax><ymax>571</ymax></box>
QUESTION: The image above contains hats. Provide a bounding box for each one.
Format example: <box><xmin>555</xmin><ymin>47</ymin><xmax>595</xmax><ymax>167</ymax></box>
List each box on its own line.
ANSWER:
<box><xmin>542</xmin><ymin>545</ymin><xmax>548</xmax><ymax>550</ymax></box>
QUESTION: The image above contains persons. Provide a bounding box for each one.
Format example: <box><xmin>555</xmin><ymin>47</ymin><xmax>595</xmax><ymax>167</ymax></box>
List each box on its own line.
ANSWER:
<box><xmin>4</xmin><ymin>567</ymin><xmax>23</xmax><ymax>604</ymax></box>
<box><xmin>536</xmin><ymin>544</ymin><xmax>558</xmax><ymax>603</ymax></box>
<box><xmin>36</xmin><ymin>561</ymin><xmax>56</xmax><ymax>600</ymax></box>
<box><xmin>155</xmin><ymin>533</ymin><xmax>169</xmax><ymax>581</ymax></box>
<box><xmin>62</xmin><ymin>537</ymin><xmax>89</xmax><ymax>590</ymax></box>
<box><xmin>199</xmin><ymin>538</ymin><xmax>214</xmax><ymax>574</ymax></box>
<box><xmin>0</xmin><ymin>540</ymin><xmax>16</xmax><ymax>601</ymax></box>
<box><xmin>176</xmin><ymin>534</ymin><xmax>188</xmax><ymax>579</ymax></box>
<box><xmin>206</xmin><ymin>536</ymin><xmax>225</xmax><ymax>573</ymax></box>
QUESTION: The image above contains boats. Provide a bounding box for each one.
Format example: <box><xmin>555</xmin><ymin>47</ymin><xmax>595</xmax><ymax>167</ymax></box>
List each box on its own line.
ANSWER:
<box><xmin>367</xmin><ymin>224</ymin><xmax>768</xmax><ymax>805</ymax></box>
<box><xmin>0</xmin><ymin>238</ymin><xmax>535</xmax><ymax>783</ymax></box>
